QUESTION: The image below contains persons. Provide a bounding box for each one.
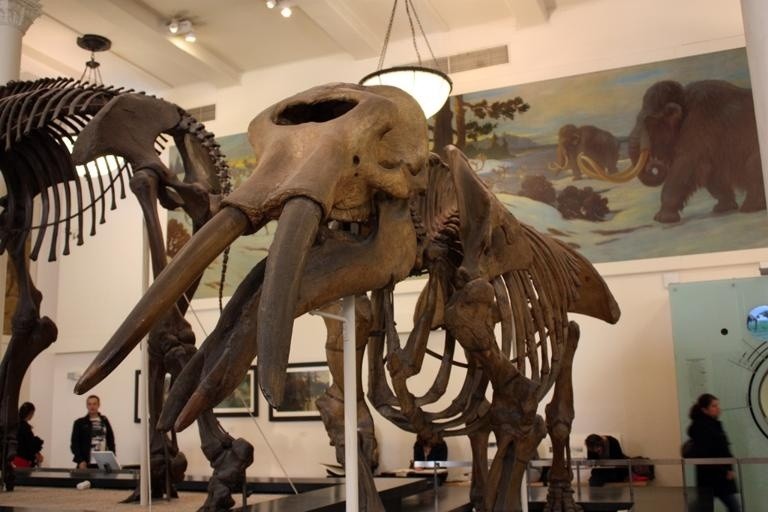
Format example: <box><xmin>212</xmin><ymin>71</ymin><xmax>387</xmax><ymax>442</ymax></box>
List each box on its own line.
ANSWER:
<box><xmin>69</xmin><ymin>394</ymin><xmax>117</xmax><ymax>471</ymax></box>
<box><xmin>583</xmin><ymin>432</ymin><xmax>635</xmax><ymax>488</ymax></box>
<box><xmin>685</xmin><ymin>391</ymin><xmax>744</xmax><ymax>512</ymax></box>
<box><xmin>10</xmin><ymin>400</ymin><xmax>44</xmax><ymax>468</ymax></box>
<box><xmin>401</xmin><ymin>434</ymin><xmax>449</xmax><ymax>489</ymax></box>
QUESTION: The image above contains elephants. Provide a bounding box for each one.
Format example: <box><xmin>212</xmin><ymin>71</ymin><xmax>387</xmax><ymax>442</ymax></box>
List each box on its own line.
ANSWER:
<box><xmin>576</xmin><ymin>78</ymin><xmax>767</xmax><ymax>225</ymax></box>
<box><xmin>547</xmin><ymin>123</ymin><xmax>622</xmax><ymax>183</ymax></box>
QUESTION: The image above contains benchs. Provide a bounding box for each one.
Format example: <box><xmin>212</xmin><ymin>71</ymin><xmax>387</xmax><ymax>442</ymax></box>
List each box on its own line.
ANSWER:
<box><xmin>444</xmin><ymin>478</ymin><xmax>652</xmax><ymax>486</ymax></box>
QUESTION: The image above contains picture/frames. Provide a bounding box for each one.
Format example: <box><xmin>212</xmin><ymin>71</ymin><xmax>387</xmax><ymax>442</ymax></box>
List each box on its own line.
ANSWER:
<box><xmin>213</xmin><ymin>367</ymin><xmax>258</xmax><ymax>419</ymax></box>
<box><xmin>147</xmin><ymin>34</ymin><xmax>768</xmax><ymax>306</ymax></box>
<box><xmin>267</xmin><ymin>360</ymin><xmax>328</xmax><ymax>420</ymax></box>
<box><xmin>134</xmin><ymin>369</ymin><xmax>144</xmax><ymax>423</ymax></box>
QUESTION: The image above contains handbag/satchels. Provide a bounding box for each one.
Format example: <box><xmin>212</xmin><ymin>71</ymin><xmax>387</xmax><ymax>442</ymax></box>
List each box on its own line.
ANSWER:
<box><xmin>681</xmin><ymin>440</ymin><xmax>700</xmax><ymax>458</ymax></box>
<box><xmin>631</xmin><ymin>456</ymin><xmax>654</xmax><ymax>476</ymax></box>
<box><xmin>13</xmin><ymin>456</ymin><xmax>33</xmax><ymax>469</ymax></box>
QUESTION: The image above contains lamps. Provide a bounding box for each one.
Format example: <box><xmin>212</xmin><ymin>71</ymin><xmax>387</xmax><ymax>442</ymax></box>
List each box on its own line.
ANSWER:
<box><xmin>49</xmin><ymin>34</ymin><xmax>126</xmax><ymax>178</ymax></box>
<box><xmin>355</xmin><ymin>0</ymin><xmax>453</xmax><ymax>121</ymax></box>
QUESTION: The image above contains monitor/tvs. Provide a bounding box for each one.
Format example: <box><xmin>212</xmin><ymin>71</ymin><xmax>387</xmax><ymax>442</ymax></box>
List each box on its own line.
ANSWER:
<box><xmin>91</xmin><ymin>451</ymin><xmax>122</xmax><ymax>472</ymax></box>
